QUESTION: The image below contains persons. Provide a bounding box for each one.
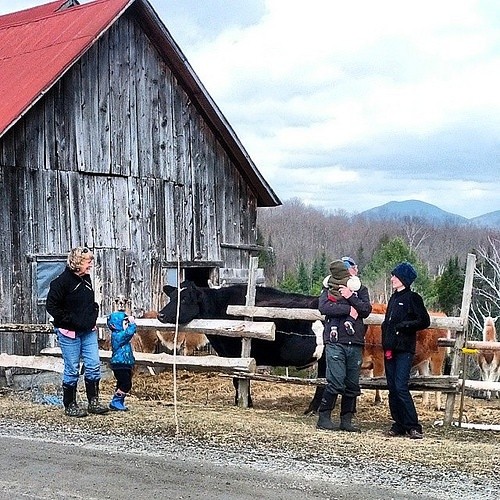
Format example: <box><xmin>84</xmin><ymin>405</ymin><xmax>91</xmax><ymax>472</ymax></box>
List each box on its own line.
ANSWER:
<box><xmin>322</xmin><ymin>259</ymin><xmax>361</xmax><ymax>343</ymax></box>
<box><xmin>381</xmin><ymin>262</ymin><xmax>431</xmax><ymax>438</ymax></box>
<box><xmin>107</xmin><ymin>312</ymin><xmax>137</xmax><ymax>412</ymax></box>
<box><xmin>46</xmin><ymin>247</ymin><xmax>109</xmax><ymax>418</ymax></box>
<box><xmin>316</xmin><ymin>257</ymin><xmax>372</xmax><ymax>434</ymax></box>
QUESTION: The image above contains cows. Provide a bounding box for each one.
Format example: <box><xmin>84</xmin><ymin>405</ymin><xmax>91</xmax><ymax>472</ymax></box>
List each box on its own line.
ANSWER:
<box><xmin>112</xmin><ymin>303</ymin><xmax>500</xmax><ymax>411</ymax></box>
<box><xmin>157</xmin><ymin>279</ymin><xmax>327</xmax><ymax>414</ymax></box>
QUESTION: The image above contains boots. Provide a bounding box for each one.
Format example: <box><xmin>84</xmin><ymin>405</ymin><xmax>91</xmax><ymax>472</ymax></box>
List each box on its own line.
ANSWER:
<box><xmin>340</xmin><ymin>395</ymin><xmax>359</xmax><ymax>431</ymax></box>
<box><xmin>316</xmin><ymin>390</ymin><xmax>340</xmax><ymax>430</ymax></box>
<box><xmin>62</xmin><ymin>379</ymin><xmax>87</xmax><ymax>417</ymax></box>
<box><xmin>84</xmin><ymin>376</ymin><xmax>109</xmax><ymax>413</ymax></box>
<box><xmin>110</xmin><ymin>389</ymin><xmax>128</xmax><ymax>411</ymax></box>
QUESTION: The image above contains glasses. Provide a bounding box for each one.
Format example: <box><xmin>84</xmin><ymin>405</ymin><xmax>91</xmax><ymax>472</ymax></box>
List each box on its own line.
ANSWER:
<box><xmin>81</xmin><ymin>248</ymin><xmax>88</xmax><ymax>253</ymax></box>
<box><xmin>391</xmin><ymin>272</ymin><xmax>394</xmax><ymax>276</ymax></box>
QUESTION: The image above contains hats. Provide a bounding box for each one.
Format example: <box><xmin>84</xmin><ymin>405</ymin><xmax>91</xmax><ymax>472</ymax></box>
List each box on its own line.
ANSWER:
<box><xmin>393</xmin><ymin>261</ymin><xmax>417</xmax><ymax>287</ymax></box>
<box><xmin>340</xmin><ymin>256</ymin><xmax>355</xmax><ymax>267</ymax></box>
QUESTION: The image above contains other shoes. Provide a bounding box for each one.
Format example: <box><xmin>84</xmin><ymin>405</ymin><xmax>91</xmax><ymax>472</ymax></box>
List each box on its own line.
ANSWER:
<box><xmin>382</xmin><ymin>427</ymin><xmax>407</xmax><ymax>436</ymax></box>
<box><xmin>407</xmin><ymin>426</ymin><xmax>423</xmax><ymax>439</ymax></box>
<box><xmin>330</xmin><ymin>330</ymin><xmax>338</xmax><ymax>342</ymax></box>
<box><xmin>344</xmin><ymin>321</ymin><xmax>355</xmax><ymax>335</ymax></box>
